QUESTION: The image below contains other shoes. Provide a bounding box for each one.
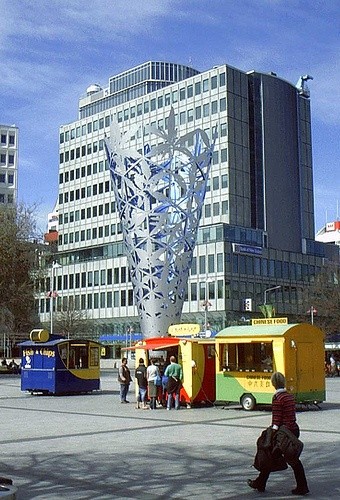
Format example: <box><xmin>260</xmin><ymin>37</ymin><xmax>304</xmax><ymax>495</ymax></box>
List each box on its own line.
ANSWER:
<box><xmin>121</xmin><ymin>401</ymin><xmax>129</xmax><ymax>403</ymax></box>
<box><xmin>247</xmin><ymin>478</ymin><xmax>266</xmax><ymax>491</ymax></box>
<box><xmin>291</xmin><ymin>485</ymin><xmax>309</xmax><ymax>494</ymax></box>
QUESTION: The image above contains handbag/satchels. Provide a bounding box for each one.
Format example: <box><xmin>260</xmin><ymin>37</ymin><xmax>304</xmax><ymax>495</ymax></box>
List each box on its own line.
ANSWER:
<box><xmin>154</xmin><ymin>366</ymin><xmax>161</xmax><ymax>386</ymax></box>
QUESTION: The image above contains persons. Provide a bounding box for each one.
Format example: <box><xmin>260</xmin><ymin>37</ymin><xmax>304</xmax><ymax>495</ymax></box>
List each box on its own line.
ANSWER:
<box><xmin>164</xmin><ymin>356</ymin><xmax>183</xmax><ymax>411</ymax></box>
<box><xmin>146</xmin><ymin>359</ymin><xmax>161</xmax><ymax>409</ymax></box>
<box><xmin>135</xmin><ymin>358</ymin><xmax>147</xmax><ymax>410</ymax></box>
<box><xmin>118</xmin><ymin>358</ymin><xmax>132</xmax><ymax>405</ymax></box>
<box><xmin>330</xmin><ymin>355</ymin><xmax>336</xmax><ymax>377</ymax></box>
<box><xmin>247</xmin><ymin>373</ymin><xmax>312</xmax><ymax>496</ymax></box>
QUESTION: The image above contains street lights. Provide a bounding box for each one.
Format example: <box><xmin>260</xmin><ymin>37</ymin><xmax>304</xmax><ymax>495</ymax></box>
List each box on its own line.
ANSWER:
<box><xmin>128</xmin><ymin>326</ymin><xmax>136</xmax><ymax>361</ymax></box>
<box><xmin>264</xmin><ymin>285</ymin><xmax>281</xmax><ymax>305</ymax></box>
<box><xmin>47</xmin><ymin>260</ymin><xmax>63</xmax><ymax>334</ymax></box>
<box><xmin>200</xmin><ymin>300</ymin><xmax>211</xmax><ymax>331</ymax></box>
<box><xmin>307</xmin><ymin>306</ymin><xmax>318</xmax><ymax>325</ymax></box>
